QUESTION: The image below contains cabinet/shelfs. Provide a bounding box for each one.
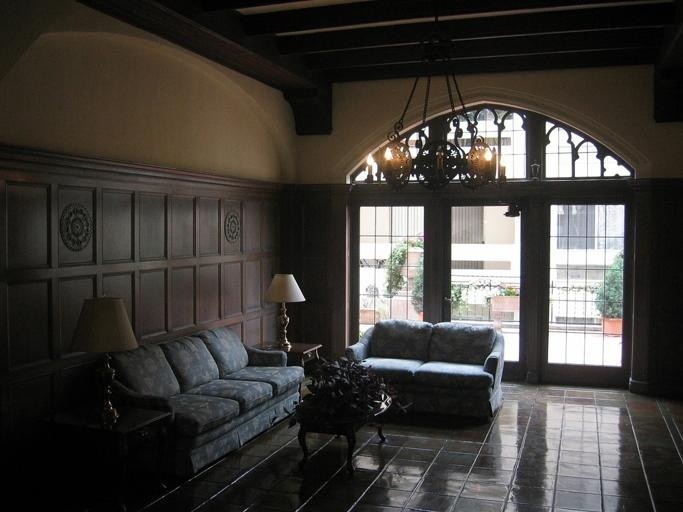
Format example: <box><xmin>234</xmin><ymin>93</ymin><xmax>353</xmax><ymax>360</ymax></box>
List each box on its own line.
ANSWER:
<box><xmin>251</xmin><ymin>341</ymin><xmax>323</xmax><ymax>405</ymax></box>
<box><xmin>33</xmin><ymin>402</ymin><xmax>172</xmax><ymax>511</ymax></box>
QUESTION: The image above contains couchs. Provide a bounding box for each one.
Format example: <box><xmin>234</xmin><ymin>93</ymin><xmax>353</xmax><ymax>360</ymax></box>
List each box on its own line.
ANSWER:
<box><xmin>344</xmin><ymin>319</ymin><xmax>505</xmax><ymax>424</ymax></box>
<box><xmin>98</xmin><ymin>326</ymin><xmax>306</xmax><ymax>479</ymax></box>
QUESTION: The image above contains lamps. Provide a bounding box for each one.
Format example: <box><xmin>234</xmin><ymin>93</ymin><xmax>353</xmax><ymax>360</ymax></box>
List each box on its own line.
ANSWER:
<box><xmin>70</xmin><ymin>292</ymin><xmax>138</xmax><ymax>426</ymax></box>
<box><xmin>365</xmin><ymin>0</ymin><xmax>508</xmax><ymax>191</ymax></box>
<box><xmin>261</xmin><ymin>274</ymin><xmax>307</xmax><ymax>350</ymax></box>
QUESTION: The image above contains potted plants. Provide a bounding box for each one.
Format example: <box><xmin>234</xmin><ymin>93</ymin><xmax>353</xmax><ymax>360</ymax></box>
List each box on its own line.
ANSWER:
<box><xmin>359</xmin><ymin>284</ymin><xmax>385</xmax><ymax>324</ymax></box>
<box><xmin>491</xmin><ymin>286</ymin><xmax>519</xmax><ymax>312</ymax></box>
<box><xmin>410</xmin><ymin>254</ymin><xmax>424</xmax><ymax>321</ymax></box>
<box><xmin>594</xmin><ymin>250</ymin><xmax>623</xmax><ymax>337</ymax></box>
